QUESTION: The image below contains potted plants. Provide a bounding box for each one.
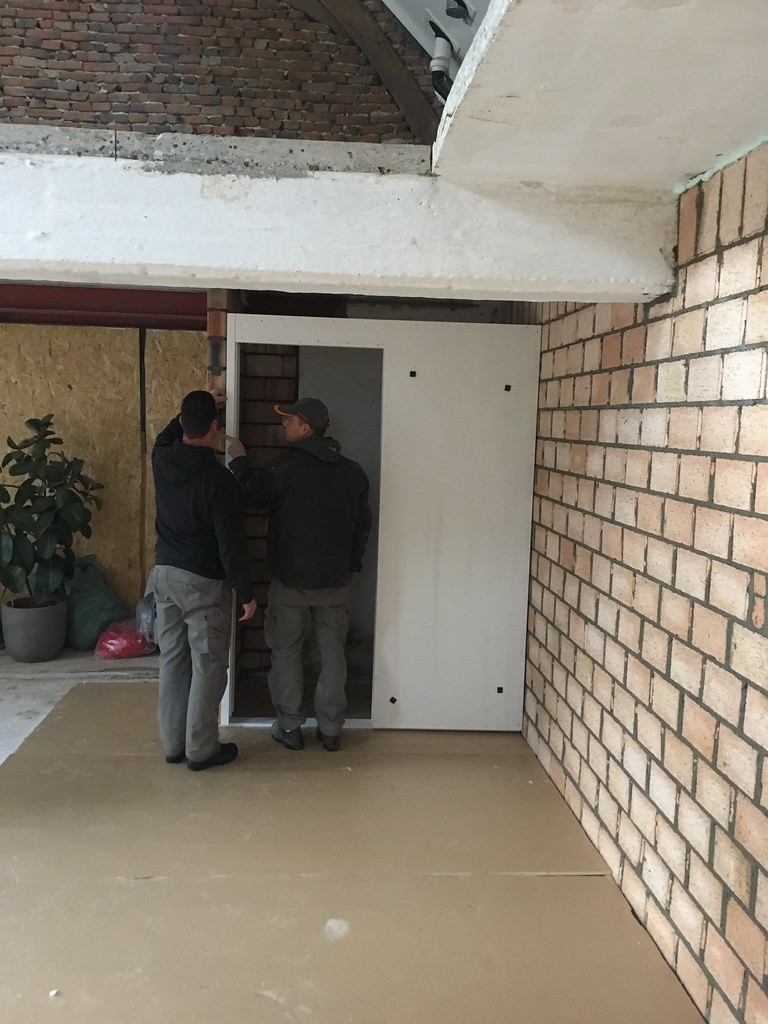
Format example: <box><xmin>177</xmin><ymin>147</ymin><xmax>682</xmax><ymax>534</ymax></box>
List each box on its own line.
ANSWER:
<box><xmin>0</xmin><ymin>414</ymin><xmax>105</xmax><ymax>663</ymax></box>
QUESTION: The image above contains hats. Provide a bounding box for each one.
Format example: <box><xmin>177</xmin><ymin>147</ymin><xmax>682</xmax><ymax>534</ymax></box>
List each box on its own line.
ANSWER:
<box><xmin>274</xmin><ymin>397</ymin><xmax>329</xmax><ymax>429</ymax></box>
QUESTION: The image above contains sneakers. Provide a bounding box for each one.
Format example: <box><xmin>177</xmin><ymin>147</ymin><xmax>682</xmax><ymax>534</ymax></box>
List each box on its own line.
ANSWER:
<box><xmin>166</xmin><ymin>753</ymin><xmax>185</xmax><ymax>764</ymax></box>
<box><xmin>316</xmin><ymin>725</ymin><xmax>340</xmax><ymax>752</ymax></box>
<box><xmin>272</xmin><ymin>720</ymin><xmax>304</xmax><ymax>751</ymax></box>
<box><xmin>186</xmin><ymin>742</ymin><xmax>239</xmax><ymax>771</ymax></box>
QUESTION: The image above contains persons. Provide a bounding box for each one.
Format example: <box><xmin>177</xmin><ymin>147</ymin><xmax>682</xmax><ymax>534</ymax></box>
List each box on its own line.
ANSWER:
<box><xmin>222</xmin><ymin>398</ymin><xmax>372</xmax><ymax>752</ymax></box>
<box><xmin>152</xmin><ymin>388</ymin><xmax>257</xmax><ymax>771</ymax></box>
